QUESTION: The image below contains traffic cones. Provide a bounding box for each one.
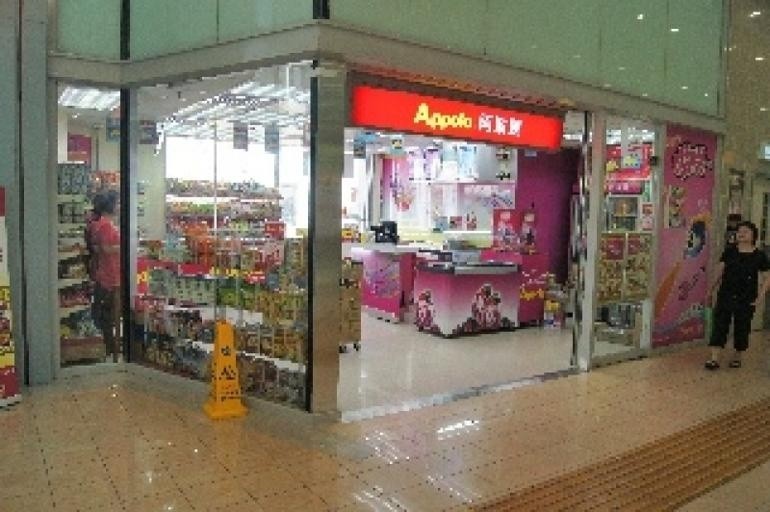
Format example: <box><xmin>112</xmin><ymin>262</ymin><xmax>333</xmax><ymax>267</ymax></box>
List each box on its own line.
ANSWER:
<box><xmin>203</xmin><ymin>318</ymin><xmax>248</xmax><ymax>420</ymax></box>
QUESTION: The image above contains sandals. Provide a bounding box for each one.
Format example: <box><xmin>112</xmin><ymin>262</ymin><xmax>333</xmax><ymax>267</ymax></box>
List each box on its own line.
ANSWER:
<box><xmin>728</xmin><ymin>360</ymin><xmax>740</xmax><ymax>368</ymax></box>
<box><xmin>705</xmin><ymin>358</ymin><xmax>718</xmax><ymax>369</ymax></box>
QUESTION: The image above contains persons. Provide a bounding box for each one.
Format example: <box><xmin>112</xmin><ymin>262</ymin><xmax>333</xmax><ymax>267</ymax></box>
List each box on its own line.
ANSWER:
<box><xmin>707</xmin><ymin>222</ymin><xmax>769</xmax><ymax>369</ymax></box>
<box><xmin>84</xmin><ymin>191</ymin><xmax>120</xmax><ymax>362</ymax></box>
